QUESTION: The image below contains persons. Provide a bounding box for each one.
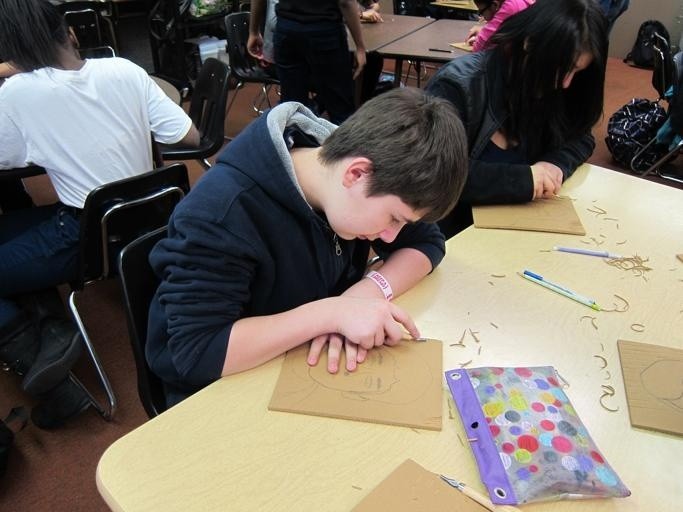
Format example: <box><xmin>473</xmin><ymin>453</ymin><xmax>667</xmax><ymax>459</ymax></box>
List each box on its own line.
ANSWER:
<box><xmin>138</xmin><ymin>82</ymin><xmax>470</xmax><ymax>412</ymax></box>
<box><xmin>670</xmin><ymin>44</ymin><xmax>682</xmax><ymax>158</ymax></box>
<box><xmin>0</xmin><ymin>1</ymin><xmax>203</xmax><ymax>431</ymax></box>
<box><xmin>0</xmin><ymin>55</ymin><xmax>22</xmax><ymax>79</ymax></box>
<box><xmin>413</xmin><ymin>1</ymin><xmax>609</xmax><ymax>243</ymax></box>
<box><xmin>464</xmin><ymin>1</ymin><xmax>536</xmax><ymax>54</ymax></box>
<box><xmin>340</xmin><ymin>2</ymin><xmax>384</xmax><ymax>96</ymax></box>
<box><xmin>246</xmin><ymin>0</ymin><xmax>368</xmax><ymax>126</ymax></box>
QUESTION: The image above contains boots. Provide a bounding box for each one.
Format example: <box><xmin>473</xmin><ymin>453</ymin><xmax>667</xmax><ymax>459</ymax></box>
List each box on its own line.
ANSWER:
<box><xmin>1</xmin><ymin>289</ymin><xmax>92</xmax><ymax>429</ymax></box>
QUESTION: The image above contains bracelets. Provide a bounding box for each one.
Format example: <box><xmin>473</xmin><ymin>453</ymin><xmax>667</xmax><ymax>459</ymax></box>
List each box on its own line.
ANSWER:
<box><xmin>361</xmin><ymin>268</ymin><xmax>395</xmax><ymax>303</ymax></box>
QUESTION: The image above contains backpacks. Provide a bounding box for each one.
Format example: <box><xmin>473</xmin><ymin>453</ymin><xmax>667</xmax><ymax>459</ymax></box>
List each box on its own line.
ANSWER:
<box><xmin>626</xmin><ymin>20</ymin><xmax>670</xmax><ymax>67</ymax></box>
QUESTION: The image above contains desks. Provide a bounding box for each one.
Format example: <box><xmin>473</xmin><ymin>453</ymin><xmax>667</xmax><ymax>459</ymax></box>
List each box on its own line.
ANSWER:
<box><xmin>1</xmin><ymin>69</ymin><xmax>183</xmax><ymax>182</ymax></box>
<box><xmin>375</xmin><ymin>19</ymin><xmax>476</xmax><ymax>90</ymax></box>
<box><xmin>92</xmin><ymin>162</ymin><xmax>682</xmax><ymax>512</ymax></box>
<box><xmin>344</xmin><ymin>11</ymin><xmax>435</xmax><ymax>106</ymax></box>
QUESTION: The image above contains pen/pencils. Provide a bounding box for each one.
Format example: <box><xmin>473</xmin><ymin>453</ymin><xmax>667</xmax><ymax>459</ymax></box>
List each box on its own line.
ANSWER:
<box><xmin>553</xmin><ymin>246</ymin><xmax>624</xmax><ymax>259</ymax></box>
<box><xmin>517</xmin><ymin>271</ymin><xmax>603</xmax><ymax>311</ymax></box>
<box><xmin>428</xmin><ymin>48</ymin><xmax>454</xmax><ymax>53</ymax></box>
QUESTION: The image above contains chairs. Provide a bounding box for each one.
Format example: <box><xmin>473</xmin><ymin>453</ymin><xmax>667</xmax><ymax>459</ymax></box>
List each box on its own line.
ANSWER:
<box><xmin>154</xmin><ymin>57</ymin><xmax>232</xmax><ymax>172</ymax></box>
<box><xmin>56</xmin><ymin>162</ymin><xmax>190</xmax><ymax>419</ymax></box>
<box><xmin>118</xmin><ymin>225</ymin><xmax>175</xmax><ymax>418</ymax></box>
<box><xmin>627</xmin><ymin>26</ymin><xmax>683</xmax><ymax>178</ymax></box>
<box><xmin>223</xmin><ymin>11</ymin><xmax>282</xmax><ymax>132</ymax></box>
<box><xmin>56</xmin><ymin>5</ymin><xmax>116</xmax><ymax>61</ymax></box>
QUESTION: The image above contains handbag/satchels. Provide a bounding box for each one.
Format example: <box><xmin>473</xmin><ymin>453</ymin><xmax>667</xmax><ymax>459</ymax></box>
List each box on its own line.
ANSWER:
<box><xmin>605</xmin><ymin>98</ymin><xmax>668</xmax><ymax>174</ymax></box>
